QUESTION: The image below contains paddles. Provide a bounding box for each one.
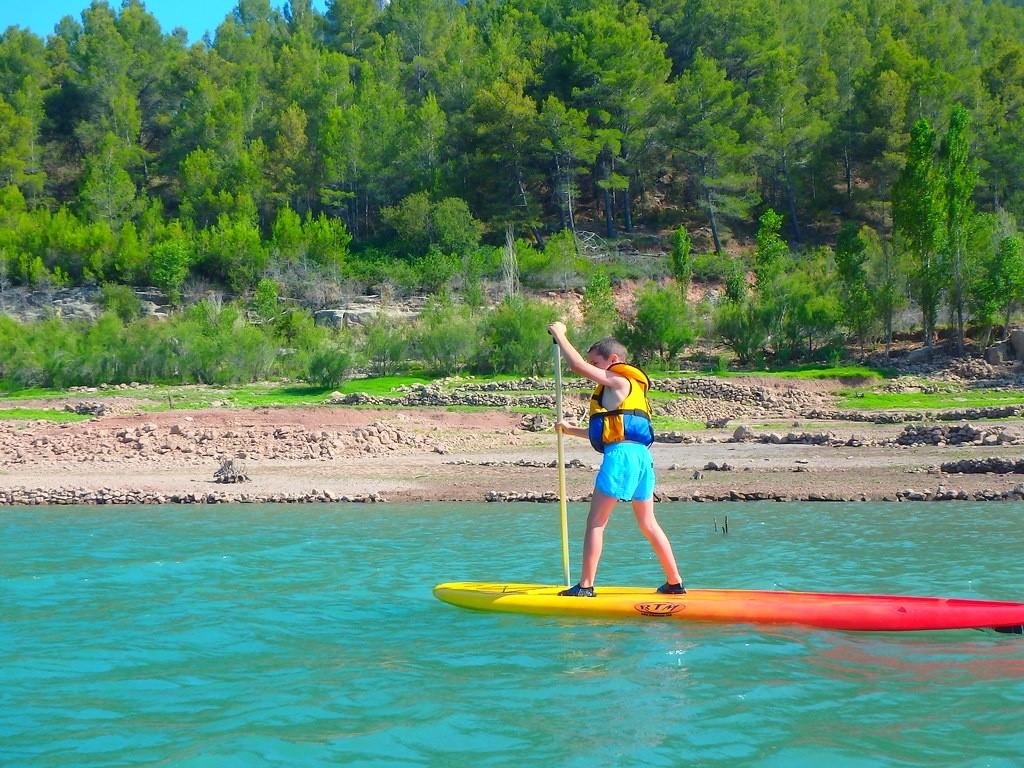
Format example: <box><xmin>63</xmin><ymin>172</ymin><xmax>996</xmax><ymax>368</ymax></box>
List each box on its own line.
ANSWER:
<box><xmin>546</xmin><ymin>329</ymin><xmax>572</xmax><ymax>587</ymax></box>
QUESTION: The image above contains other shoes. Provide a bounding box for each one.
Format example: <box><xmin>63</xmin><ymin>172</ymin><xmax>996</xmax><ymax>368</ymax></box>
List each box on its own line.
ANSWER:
<box><xmin>657</xmin><ymin>580</ymin><xmax>684</xmax><ymax>594</ymax></box>
<box><xmin>558</xmin><ymin>583</ymin><xmax>596</xmax><ymax>596</ymax></box>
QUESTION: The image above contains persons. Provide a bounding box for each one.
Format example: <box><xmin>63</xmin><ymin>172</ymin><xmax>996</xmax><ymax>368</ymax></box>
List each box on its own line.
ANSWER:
<box><xmin>546</xmin><ymin>320</ymin><xmax>687</xmax><ymax>598</ymax></box>
